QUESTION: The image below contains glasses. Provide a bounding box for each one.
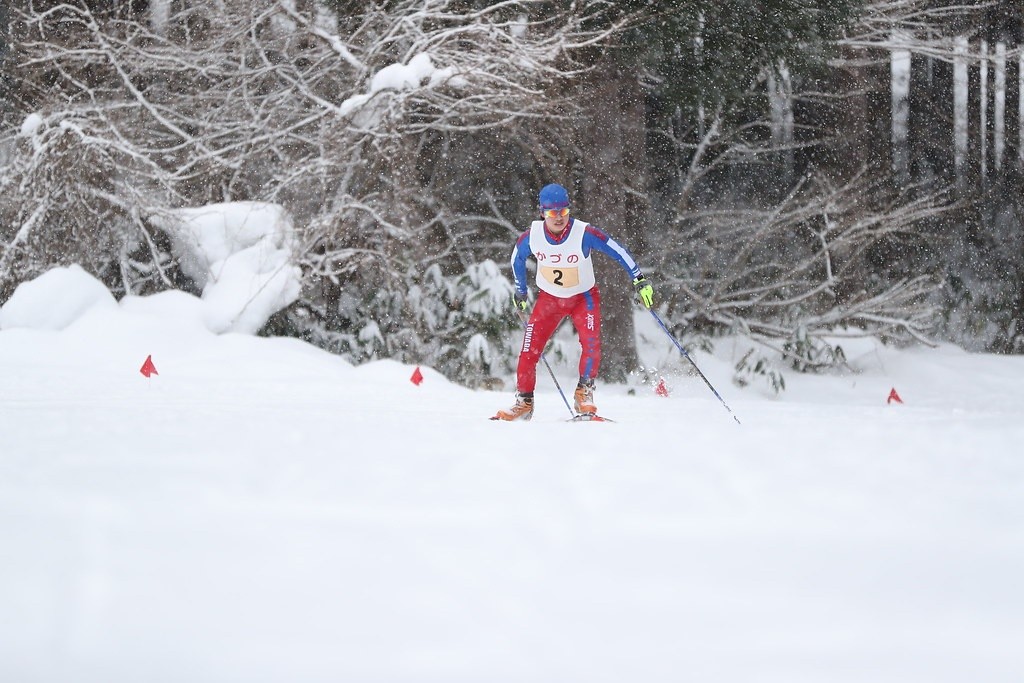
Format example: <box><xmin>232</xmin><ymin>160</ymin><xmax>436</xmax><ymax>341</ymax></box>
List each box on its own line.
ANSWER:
<box><xmin>542</xmin><ymin>208</ymin><xmax>571</xmax><ymax>218</ymax></box>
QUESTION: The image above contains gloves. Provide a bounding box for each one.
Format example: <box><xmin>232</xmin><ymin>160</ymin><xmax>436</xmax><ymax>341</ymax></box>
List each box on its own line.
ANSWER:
<box><xmin>513</xmin><ymin>292</ymin><xmax>527</xmax><ymax>310</ymax></box>
<box><xmin>633</xmin><ymin>278</ymin><xmax>653</xmax><ymax>308</ymax></box>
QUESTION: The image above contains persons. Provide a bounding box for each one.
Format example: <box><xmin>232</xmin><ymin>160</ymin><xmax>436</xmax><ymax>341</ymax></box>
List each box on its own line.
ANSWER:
<box><xmin>497</xmin><ymin>183</ymin><xmax>653</xmax><ymax>420</ymax></box>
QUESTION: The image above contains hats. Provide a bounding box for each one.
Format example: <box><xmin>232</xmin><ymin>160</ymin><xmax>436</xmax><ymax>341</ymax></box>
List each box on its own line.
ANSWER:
<box><xmin>540</xmin><ymin>184</ymin><xmax>569</xmax><ymax>210</ymax></box>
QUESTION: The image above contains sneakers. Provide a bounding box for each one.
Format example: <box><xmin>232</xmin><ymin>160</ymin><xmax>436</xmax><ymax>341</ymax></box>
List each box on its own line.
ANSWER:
<box><xmin>574</xmin><ymin>383</ymin><xmax>596</xmax><ymax>414</ymax></box>
<box><xmin>497</xmin><ymin>396</ymin><xmax>535</xmax><ymax>421</ymax></box>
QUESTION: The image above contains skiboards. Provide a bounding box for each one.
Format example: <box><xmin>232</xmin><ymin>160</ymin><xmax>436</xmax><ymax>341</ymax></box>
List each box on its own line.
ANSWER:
<box><xmin>488</xmin><ymin>412</ymin><xmax>613</xmax><ymax>423</ymax></box>
